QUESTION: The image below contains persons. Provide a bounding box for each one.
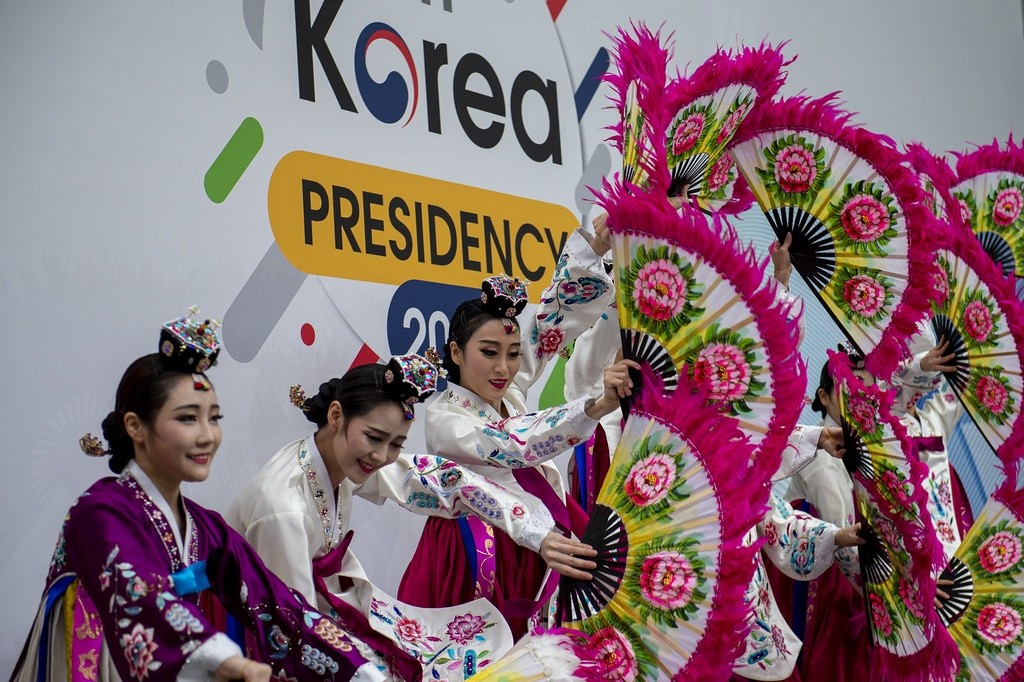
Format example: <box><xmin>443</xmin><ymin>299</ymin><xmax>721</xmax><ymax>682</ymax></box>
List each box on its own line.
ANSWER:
<box><xmin>564</xmin><ymin>182</ymin><xmax>966</xmax><ymax>682</ymax></box>
<box><xmin>10</xmin><ymin>304</ymin><xmax>390</xmax><ymax>682</ymax></box>
<box><xmin>197</xmin><ymin>354</ymin><xmax>598</xmax><ymax>682</ymax></box>
<box><xmin>396</xmin><ymin>212</ymin><xmax>642</xmax><ymax>644</ymax></box>
